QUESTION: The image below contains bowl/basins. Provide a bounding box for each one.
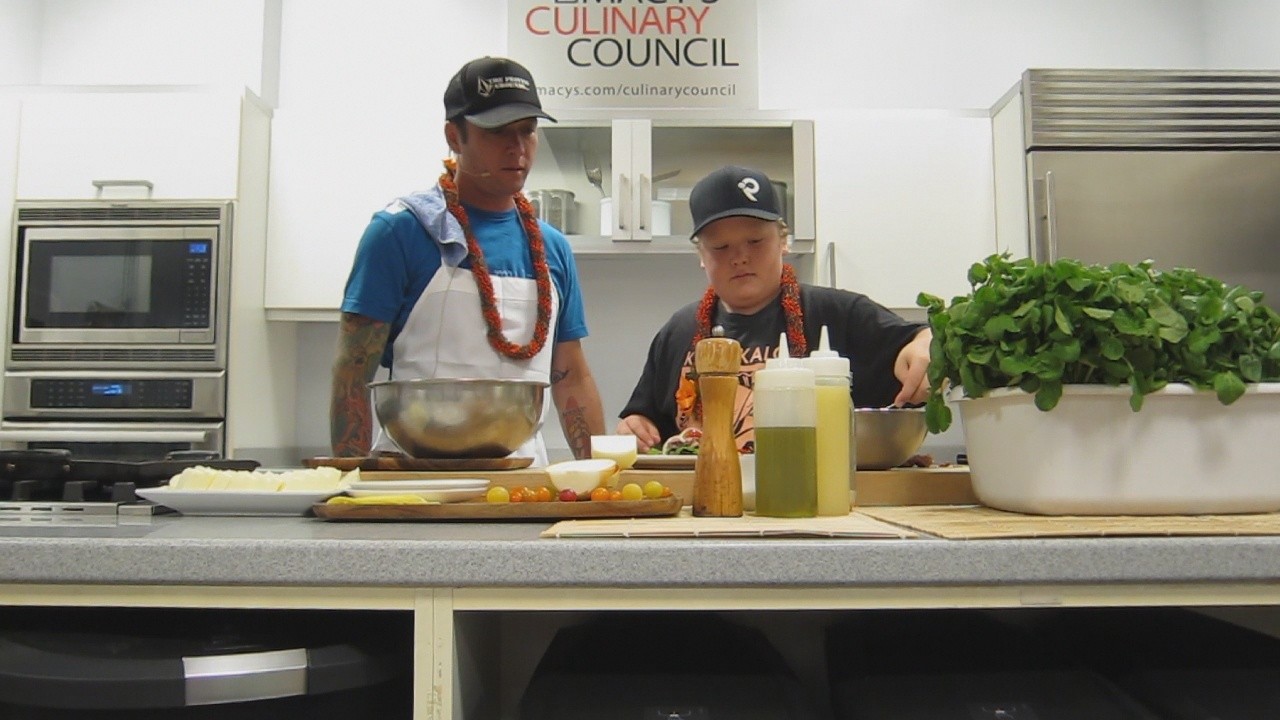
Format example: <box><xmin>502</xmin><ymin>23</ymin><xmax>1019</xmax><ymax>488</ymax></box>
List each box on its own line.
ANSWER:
<box><xmin>854</xmin><ymin>408</ymin><xmax>929</xmax><ymax>471</ymax></box>
<box><xmin>365</xmin><ymin>375</ymin><xmax>550</xmax><ymax>459</ymax></box>
<box><xmin>546</xmin><ymin>458</ymin><xmax>616</xmax><ymax>499</ymax></box>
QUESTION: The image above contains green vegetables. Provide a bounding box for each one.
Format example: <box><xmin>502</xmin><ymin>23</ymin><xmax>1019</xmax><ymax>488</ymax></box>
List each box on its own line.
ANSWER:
<box><xmin>915</xmin><ymin>249</ymin><xmax>1280</xmax><ymax>435</ymax></box>
<box><xmin>647</xmin><ymin>446</ymin><xmax>700</xmax><ymax>455</ymax></box>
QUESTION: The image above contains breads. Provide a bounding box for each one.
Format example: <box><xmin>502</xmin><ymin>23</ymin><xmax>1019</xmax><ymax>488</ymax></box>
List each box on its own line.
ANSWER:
<box><xmin>169</xmin><ymin>465</ymin><xmax>342</xmax><ymax>490</ymax></box>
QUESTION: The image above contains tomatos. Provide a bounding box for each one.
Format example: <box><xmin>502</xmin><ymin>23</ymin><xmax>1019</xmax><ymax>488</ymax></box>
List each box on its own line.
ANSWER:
<box><xmin>559</xmin><ymin>488</ymin><xmax>577</xmax><ymax>501</ymax></box>
<box><xmin>487</xmin><ymin>486</ymin><xmax>551</xmax><ymax>503</ymax></box>
<box><xmin>591</xmin><ymin>481</ymin><xmax>670</xmax><ymax>501</ymax></box>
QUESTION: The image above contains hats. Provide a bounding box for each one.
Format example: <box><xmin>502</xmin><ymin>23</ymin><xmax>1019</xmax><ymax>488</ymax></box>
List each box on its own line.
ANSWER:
<box><xmin>687</xmin><ymin>164</ymin><xmax>782</xmax><ymax>240</ymax></box>
<box><xmin>443</xmin><ymin>55</ymin><xmax>559</xmax><ymax>128</ymax></box>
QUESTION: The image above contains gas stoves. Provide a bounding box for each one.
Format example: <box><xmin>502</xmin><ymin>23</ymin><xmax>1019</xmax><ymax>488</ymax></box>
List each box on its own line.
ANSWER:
<box><xmin>0</xmin><ymin>449</ymin><xmax>261</xmax><ymax>515</ymax></box>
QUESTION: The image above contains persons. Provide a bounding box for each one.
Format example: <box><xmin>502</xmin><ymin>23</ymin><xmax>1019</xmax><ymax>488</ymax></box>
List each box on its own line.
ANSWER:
<box><xmin>329</xmin><ymin>58</ymin><xmax>606</xmax><ymax>467</ymax></box>
<box><xmin>618</xmin><ymin>168</ymin><xmax>947</xmax><ymax>456</ymax></box>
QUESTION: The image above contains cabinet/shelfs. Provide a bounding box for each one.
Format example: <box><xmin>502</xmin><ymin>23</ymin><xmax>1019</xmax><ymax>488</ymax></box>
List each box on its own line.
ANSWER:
<box><xmin>515</xmin><ymin>115</ymin><xmax>815</xmax><ymax>253</ymax></box>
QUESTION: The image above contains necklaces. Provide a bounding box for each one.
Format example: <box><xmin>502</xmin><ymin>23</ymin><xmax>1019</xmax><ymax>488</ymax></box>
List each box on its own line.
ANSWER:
<box><xmin>690</xmin><ymin>263</ymin><xmax>805</xmax><ymax>454</ymax></box>
<box><xmin>440</xmin><ymin>159</ymin><xmax>552</xmax><ymax>360</ymax></box>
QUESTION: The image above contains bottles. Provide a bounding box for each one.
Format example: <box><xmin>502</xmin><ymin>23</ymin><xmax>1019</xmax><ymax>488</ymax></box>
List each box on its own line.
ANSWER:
<box><xmin>752</xmin><ymin>331</ymin><xmax>819</xmax><ymax>516</ymax></box>
<box><xmin>809</xmin><ymin>323</ymin><xmax>852</xmax><ymax>516</ymax></box>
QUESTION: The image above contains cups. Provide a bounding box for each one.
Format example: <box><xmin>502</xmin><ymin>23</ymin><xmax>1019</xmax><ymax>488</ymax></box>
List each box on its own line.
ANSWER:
<box><xmin>591</xmin><ymin>433</ymin><xmax>638</xmax><ymax>470</ymax></box>
<box><xmin>651</xmin><ymin>200</ymin><xmax>672</xmax><ymax>237</ymax></box>
<box><xmin>600</xmin><ymin>197</ymin><xmax>616</xmax><ymax>236</ymax></box>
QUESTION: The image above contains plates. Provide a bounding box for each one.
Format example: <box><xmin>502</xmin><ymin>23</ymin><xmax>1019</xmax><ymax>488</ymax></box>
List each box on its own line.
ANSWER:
<box><xmin>131</xmin><ymin>484</ymin><xmax>344</xmax><ymax>516</ymax></box>
<box><xmin>345</xmin><ymin>479</ymin><xmax>489</xmax><ymax>502</ymax></box>
<box><xmin>633</xmin><ymin>454</ymin><xmax>699</xmax><ymax>471</ymax></box>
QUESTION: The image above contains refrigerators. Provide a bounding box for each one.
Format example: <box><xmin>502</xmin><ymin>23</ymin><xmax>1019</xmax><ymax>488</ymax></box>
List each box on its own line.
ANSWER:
<box><xmin>988</xmin><ymin>67</ymin><xmax>1280</xmax><ymax>314</ymax></box>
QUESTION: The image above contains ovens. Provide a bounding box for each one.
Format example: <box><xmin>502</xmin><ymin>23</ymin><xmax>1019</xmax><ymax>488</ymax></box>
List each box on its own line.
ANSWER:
<box><xmin>0</xmin><ymin>371</ymin><xmax>226</xmax><ymax>458</ymax></box>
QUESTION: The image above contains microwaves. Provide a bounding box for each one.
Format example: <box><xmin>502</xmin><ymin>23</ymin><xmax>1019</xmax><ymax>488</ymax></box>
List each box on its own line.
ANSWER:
<box><xmin>19</xmin><ymin>202</ymin><xmax>232</xmax><ymax>346</ymax></box>
